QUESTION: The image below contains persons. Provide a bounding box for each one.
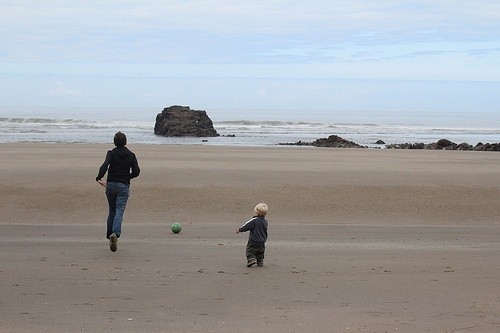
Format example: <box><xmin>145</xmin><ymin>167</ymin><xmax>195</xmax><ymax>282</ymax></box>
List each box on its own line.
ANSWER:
<box><xmin>236</xmin><ymin>202</ymin><xmax>268</xmax><ymax>268</ymax></box>
<box><xmin>96</xmin><ymin>131</ymin><xmax>140</xmax><ymax>252</ymax></box>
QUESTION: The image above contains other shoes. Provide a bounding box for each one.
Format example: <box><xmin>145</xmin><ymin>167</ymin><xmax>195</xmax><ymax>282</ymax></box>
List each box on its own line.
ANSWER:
<box><xmin>257</xmin><ymin>260</ymin><xmax>263</xmax><ymax>266</ymax></box>
<box><xmin>110</xmin><ymin>232</ymin><xmax>119</xmax><ymax>252</ymax></box>
<box><xmin>247</xmin><ymin>258</ymin><xmax>256</xmax><ymax>266</ymax></box>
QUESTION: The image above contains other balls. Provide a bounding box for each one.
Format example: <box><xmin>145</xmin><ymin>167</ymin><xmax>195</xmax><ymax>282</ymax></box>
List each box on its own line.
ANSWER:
<box><xmin>171</xmin><ymin>222</ymin><xmax>181</xmax><ymax>234</ymax></box>
